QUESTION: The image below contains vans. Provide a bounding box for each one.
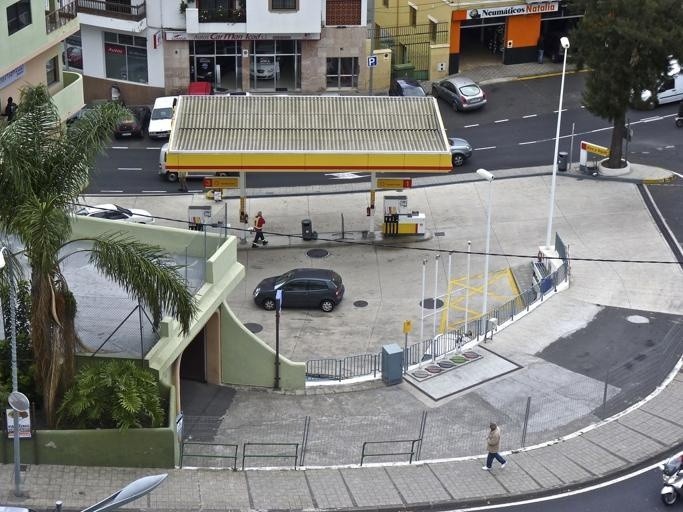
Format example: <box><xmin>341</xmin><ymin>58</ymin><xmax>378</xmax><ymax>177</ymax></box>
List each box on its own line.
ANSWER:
<box><xmin>158</xmin><ymin>143</ymin><xmax>234</xmax><ymax>182</ymax></box>
<box><xmin>627</xmin><ymin>71</ymin><xmax>682</xmax><ymax>110</ymax></box>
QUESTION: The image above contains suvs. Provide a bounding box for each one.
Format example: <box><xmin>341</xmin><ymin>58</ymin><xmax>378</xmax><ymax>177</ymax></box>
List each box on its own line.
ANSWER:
<box><xmin>112</xmin><ymin>104</ymin><xmax>149</xmax><ymax>139</ymax></box>
<box><xmin>253</xmin><ymin>267</ymin><xmax>343</xmax><ymax>312</ymax></box>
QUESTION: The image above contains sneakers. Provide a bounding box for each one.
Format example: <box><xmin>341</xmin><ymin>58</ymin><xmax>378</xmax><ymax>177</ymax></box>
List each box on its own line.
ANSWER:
<box><xmin>263</xmin><ymin>241</ymin><xmax>268</xmax><ymax>245</ymax></box>
<box><xmin>251</xmin><ymin>244</ymin><xmax>259</xmax><ymax>248</ymax></box>
<box><xmin>500</xmin><ymin>460</ymin><xmax>507</xmax><ymax>469</ymax></box>
<box><xmin>481</xmin><ymin>466</ymin><xmax>492</xmax><ymax>470</ymax></box>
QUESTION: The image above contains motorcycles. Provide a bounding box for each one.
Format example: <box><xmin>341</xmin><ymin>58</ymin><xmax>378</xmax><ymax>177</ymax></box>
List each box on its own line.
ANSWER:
<box><xmin>675</xmin><ymin>116</ymin><xmax>683</xmax><ymax>127</ymax></box>
<box><xmin>657</xmin><ymin>452</ymin><xmax>683</xmax><ymax>504</ymax></box>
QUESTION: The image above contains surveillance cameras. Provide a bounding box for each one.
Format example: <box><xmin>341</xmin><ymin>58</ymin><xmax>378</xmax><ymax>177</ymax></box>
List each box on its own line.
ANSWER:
<box><xmin>476</xmin><ymin>168</ymin><xmax>495</xmax><ymax>182</ymax></box>
<box><xmin>560</xmin><ymin>37</ymin><xmax>570</xmax><ymax>49</ymax></box>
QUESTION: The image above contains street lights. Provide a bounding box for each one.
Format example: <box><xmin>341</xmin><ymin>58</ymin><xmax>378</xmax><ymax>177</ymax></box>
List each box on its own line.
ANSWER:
<box><xmin>546</xmin><ymin>37</ymin><xmax>570</xmax><ymax>252</ymax></box>
<box><xmin>0</xmin><ymin>246</ymin><xmax>22</xmax><ymax>495</ymax></box>
<box><xmin>78</xmin><ymin>472</ymin><xmax>170</xmax><ymax>512</ymax></box>
<box><xmin>476</xmin><ymin>168</ymin><xmax>493</xmax><ymax>335</ymax></box>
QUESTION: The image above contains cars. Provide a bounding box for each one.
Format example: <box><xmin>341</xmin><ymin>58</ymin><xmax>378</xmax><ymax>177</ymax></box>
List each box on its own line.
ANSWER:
<box><xmin>432</xmin><ymin>75</ymin><xmax>488</xmax><ymax>114</ymax></box>
<box><xmin>448</xmin><ymin>137</ymin><xmax>471</xmax><ymax>165</ymax></box>
<box><xmin>61</xmin><ymin>47</ymin><xmax>81</xmax><ymax>68</ymax></box>
<box><xmin>147</xmin><ymin>95</ymin><xmax>179</xmax><ymax>138</ymax></box>
<box><xmin>389</xmin><ymin>79</ymin><xmax>425</xmax><ymax>97</ymax></box>
<box><xmin>75</xmin><ymin>203</ymin><xmax>154</xmax><ymax>225</ymax></box>
<box><xmin>249</xmin><ymin>57</ymin><xmax>280</xmax><ymax>80</ymax></box>
<box><xmin>190</xmin><ymin>45</ymin><xmax>222</xmax><ymax>82</ymax></box>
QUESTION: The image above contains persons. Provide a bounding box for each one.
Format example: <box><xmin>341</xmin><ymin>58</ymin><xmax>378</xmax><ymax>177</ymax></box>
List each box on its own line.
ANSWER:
<box><xmin>1</xmin><ymin>96</ymin><xmax>18</xmax><ymax>121</ymax></box>
<box><xmin>252</xmin><ymin>211</ymin><xmax>268</xmax><ymax>248</ymax></box>
<box><xmin>178</xmin><ymin>172</ymin><xmax>188</xmax><ymax>192</ymax></box>
<box><xmin>481</xmin><ymin>422</ymin><xmax>507</xmax><ymax>470</ymax></box>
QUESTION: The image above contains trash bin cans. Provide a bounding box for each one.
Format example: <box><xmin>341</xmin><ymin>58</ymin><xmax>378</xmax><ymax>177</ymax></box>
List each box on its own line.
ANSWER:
<box><xmin>536</xmin><ymin>48</ymin><xmax>544</xmax><ymax>65</ymax></box>
<box><xmin>301</xmin><ymin>219</ymin><xmax>313</xmax><ymax>241</ymax></box>
<box><xmin>557</xmin><ymin>151</ymin><xmax>568</xmax><ymax>172</ymax></box>
